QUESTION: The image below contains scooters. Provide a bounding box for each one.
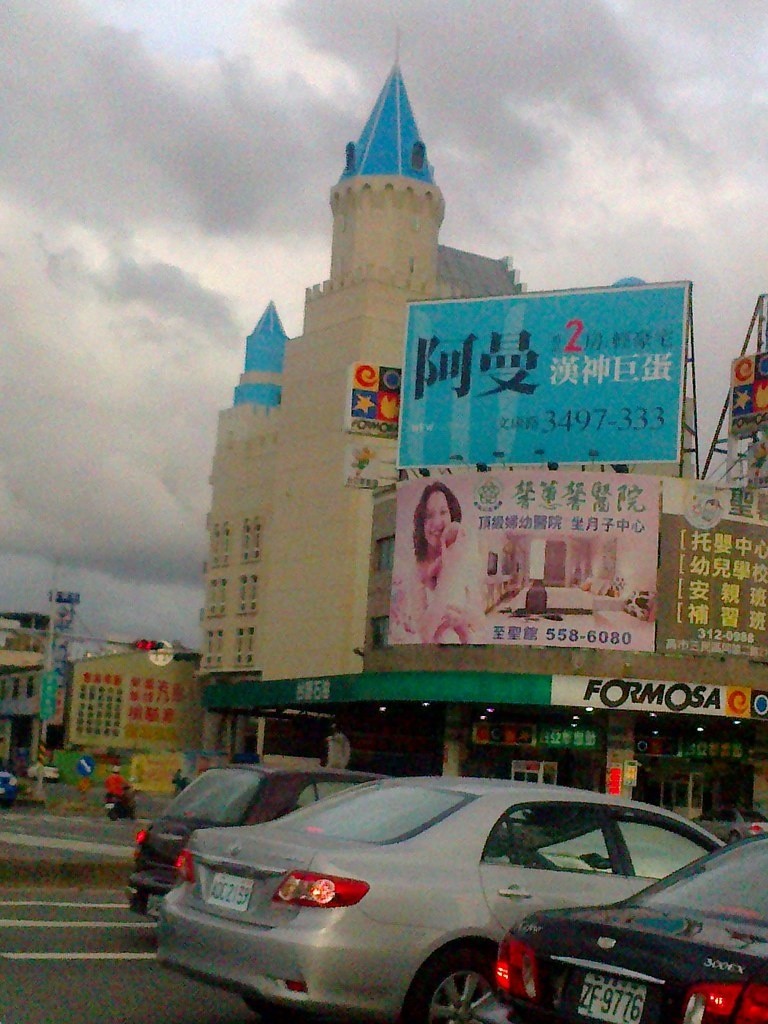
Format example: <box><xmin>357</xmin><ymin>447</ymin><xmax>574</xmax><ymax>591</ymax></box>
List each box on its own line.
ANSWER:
<box><xmin>104</xmin><ymin>789</ymin><xmax>134</xmax><ymax>821</ymax></box>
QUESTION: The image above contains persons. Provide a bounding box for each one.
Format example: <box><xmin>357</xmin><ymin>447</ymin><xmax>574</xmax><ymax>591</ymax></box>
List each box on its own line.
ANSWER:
<box><xmin>410</xmin><ymin>480</ymin><xmax>487</xmax><ymax>645</ymax></box>
<box><xmin>171</xmin><ymin>769</ymin><xmax>184</xmax><ymax>793</ymax></box>
<box><xmin>104</xmin><ymin>766</ymin><xmax>137</xmax><ymax>820</ymax></box>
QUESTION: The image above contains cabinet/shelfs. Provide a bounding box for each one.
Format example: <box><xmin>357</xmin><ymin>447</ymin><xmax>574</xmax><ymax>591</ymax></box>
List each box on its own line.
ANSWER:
<box><xmin>511</xmin><ymin>760</ymin><xmax>557</xmax><ymax>786</ymax></box>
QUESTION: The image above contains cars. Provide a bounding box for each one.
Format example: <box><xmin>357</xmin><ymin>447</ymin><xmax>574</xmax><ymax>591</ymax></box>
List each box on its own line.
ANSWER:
<box><xmin>122</xmin><ymin>765</ymin><xmax>388</xmax><ymax>915</ymax></box>
<box><xmin>158</xmin><ymin>776</ymin><xmax>729</xmax><ymax>1024</ymax></box>
<box><xmin>0</xmin><ymin>768</ymin><xmax>17</xmax><ymax>805</ymax></box>
<box><xmin>498</xmin><ymin>833</ymin><xmax>767</xmax><ymax>1022</ymax></box>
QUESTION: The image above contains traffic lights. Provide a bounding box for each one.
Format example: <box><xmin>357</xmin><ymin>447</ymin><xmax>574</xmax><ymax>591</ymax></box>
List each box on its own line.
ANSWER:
<box><xmin>129</xmin><ymin>640</ymin><xmax>161</xmax><ymax>652</ymax></box>
